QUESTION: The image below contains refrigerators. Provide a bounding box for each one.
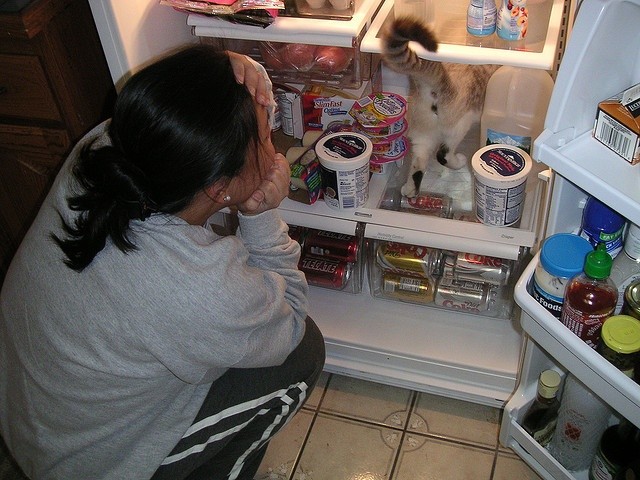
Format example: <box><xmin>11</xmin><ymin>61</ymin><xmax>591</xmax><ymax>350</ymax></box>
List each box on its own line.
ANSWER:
<box><xmin>89</xmin><ymin>1</ymin><xmax>639</xmax><ymax>480</ymax></box>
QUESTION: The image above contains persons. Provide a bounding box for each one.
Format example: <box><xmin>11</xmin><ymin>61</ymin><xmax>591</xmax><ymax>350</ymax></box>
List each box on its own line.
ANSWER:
<box><xmin>0</xmin><ymin>44</ymin><xmax>325</xmax><ymax>480</ymax></box>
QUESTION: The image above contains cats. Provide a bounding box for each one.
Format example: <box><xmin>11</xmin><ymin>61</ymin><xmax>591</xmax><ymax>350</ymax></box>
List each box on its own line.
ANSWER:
<box><xmin>382</xmin><ymin>14</ymin><xmax>503</xmax><ymax>201</ymax></box>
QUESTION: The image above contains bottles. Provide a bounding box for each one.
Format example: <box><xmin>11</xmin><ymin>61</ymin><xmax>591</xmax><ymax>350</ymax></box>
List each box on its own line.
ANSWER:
<box><xmin>589</xmin><ymin>423</ymin><xmax>640</xmax><ymax>480</ymax></box>
<box><xmin>532</xmin><ymin>232</ymin><xmax>594</xmax><ymax>319</ymax></box>
<box><xmin>600</xmin><ymin>315</ymin><xmax>640</xmax><ymax>379</ymax></box>
<box><xmin>466</xmin><ymin>0</ymin><xmax>497</xmax><ymax>38</ymax></box>
<box><xmin>580</xmin><ymin>195</ymin><xmax>625</xmax><ymax>261</ymax></box>
<box><xmin>496</xmin><ymin>0</ymin><xmax>529</xmax><ymax>40</ymax></box>
<box><xmin>549</xmin><ymin>372</ymin><xmax>614</xmax><ymax>471</ymax></box>
<box><xmin>522</xmin><ymin>369</ymin><xmax>562</xmax><ymax>448</ymax></box>
<box><xmin>623</xmin><ymin>222</ymin><xmax>640</xmax><ymax>261</ymax></box>
<box><xmin>561</xmin><ymin>242</ymin><xmax>619</xmax><ymax>351</ymax></box>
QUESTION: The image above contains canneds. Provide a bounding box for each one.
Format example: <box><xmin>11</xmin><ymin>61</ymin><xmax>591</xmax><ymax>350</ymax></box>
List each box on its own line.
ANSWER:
<box><xmin>376</xmin><ymin>241</ymin><xmax>439</xmax><ymax>279</ymax></box>
<box><xmin>444</xmin><ymin>251</ymin><xmax>510</xmax><ymax>286</ymax></box>
<box><xmin>302</xmin><ymin>229</ymin><xmax>359</xmax><ymax>262</ymax></box>
<box><xmin>401</xmin><ymin>193</ymin><xmax>454</xmax><ymax>219</ymax></box>
<box><xmin>384</xmin><ymin>273</ymin><xmax>434</xmax><ymax>303</ymax></box>
<box><xmin>435</xmin><ymin>277</ymin><xmax>497</xmax><ymax>312</ymax></box>
<box><xmin>298</xmin><ymin>256</ymin><xmax>352</xmax><ymax>290</ymax></box>
